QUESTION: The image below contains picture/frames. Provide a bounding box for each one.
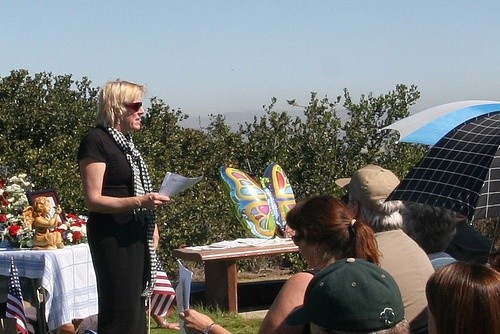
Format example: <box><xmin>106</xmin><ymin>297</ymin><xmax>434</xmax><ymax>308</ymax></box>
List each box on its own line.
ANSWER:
<box><xmin>28</xmin><ymin>189</ymin><xmax>67</xmax><ymax>225</ymax></box>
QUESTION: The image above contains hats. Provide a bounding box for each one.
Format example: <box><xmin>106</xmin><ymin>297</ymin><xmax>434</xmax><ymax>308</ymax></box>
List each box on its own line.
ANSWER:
<box><xmin>286</xmin><ymin>257</ymin><xmax>405</xmax><ymax>331</ymax></box>
<box><xmin>334</xmin><ymin>164</ymin><xmax>401</xmax><ymax>213</ymax></box>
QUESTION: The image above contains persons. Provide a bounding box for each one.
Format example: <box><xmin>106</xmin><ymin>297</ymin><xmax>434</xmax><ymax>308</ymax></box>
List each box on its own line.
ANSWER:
<box><xmin>24</xmin><ymin>304</ymin><xmax>181</xmax><ymax>334</ymax></box>
<box><xmin>425</xmin><ymin>260</ymin><xmax>500</xmax><ymax>334</ymax></box>
<box><xmin>400</xmin><ymin>201</ymin><xmax>457</xmax><ymax>271</ymax></box>
<box><xmin>335</xmin><ymin>164</ymin><xmax>436</xmax><ymax>334</ymax></box>
<box><xmin>443</xmin><ymin>222</ymin><xmax>491</xmax><ymax>265</ymax></box>
<box><xmin>78</xmin><ymin>80</ymin><xmax>171</xmax><ymax>334</ymax></box>
<box><xmin>22</xmin><ymin>196</ymin><xmax>65</xmax><ymax>249</ymax></box>
<box><xmin>178</xmin><ymin>195</ymin><xmax>382</xmax><ymax>334</ymax></box>
<box><xmin>287</xmin><ymin>257</ymin><xmax>410</xmax><ymax>334</ymax></box>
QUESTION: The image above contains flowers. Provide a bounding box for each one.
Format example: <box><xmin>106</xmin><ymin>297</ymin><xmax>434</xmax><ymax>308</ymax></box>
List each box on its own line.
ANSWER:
<box><xmin>0</xmin><ymin>172</ymin><xmax>35</xmax><ymax>249</ymax></box>
<box><xmin>59</xmin><ymin>211</ymin><xmax>88</xmax><ymax>244</ymax></box>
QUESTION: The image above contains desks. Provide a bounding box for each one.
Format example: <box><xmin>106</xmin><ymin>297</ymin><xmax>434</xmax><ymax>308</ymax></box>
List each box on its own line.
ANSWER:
<box><xmin>0</xmin><ymin>247</ymin><xmax>98</xmax><ymax>334</ymax></box>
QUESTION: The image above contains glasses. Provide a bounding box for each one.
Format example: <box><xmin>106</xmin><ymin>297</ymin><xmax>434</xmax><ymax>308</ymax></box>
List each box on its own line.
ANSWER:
<box><xmin>292</xmin><ymin>233</ymin><xmax>310</xmax><ymax>247</ymax></box>
<box><xmin>123</xmin><ymin>102</ymin><xmax>142</xmax><ymax>111</ymax></box>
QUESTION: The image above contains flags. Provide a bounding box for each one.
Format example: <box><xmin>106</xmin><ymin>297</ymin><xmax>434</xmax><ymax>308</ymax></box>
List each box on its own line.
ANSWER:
<box><xmin>147</xmin><ymin>255</ymin><xmax>178</xmax><ymax>319</ymax></box>
<box><xmin>5</xmin><ymin>258</ymin><xmax>29</xmax><ymax>334</ymax></box>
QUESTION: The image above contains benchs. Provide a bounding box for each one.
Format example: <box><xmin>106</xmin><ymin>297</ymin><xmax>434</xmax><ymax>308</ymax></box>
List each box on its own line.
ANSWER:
<box><xmin>174</xmin><ymin>240</ymin><xmax>300</xmax><ymax>314</ymax></box>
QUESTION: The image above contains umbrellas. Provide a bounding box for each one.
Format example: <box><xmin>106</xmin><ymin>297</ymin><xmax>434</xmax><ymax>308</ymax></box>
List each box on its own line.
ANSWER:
<box><xmin>380</xmin><ymin>99</ymin><xmax>500</xmax><ymax>226</ymax></box>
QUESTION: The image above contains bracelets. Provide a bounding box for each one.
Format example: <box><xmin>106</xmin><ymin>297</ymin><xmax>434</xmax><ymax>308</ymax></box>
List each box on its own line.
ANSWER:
<box><xmin>157</xmin><ymin>319</ymin><xmax>167</xmax><ymax>328</ymax></box>
<box><xmin>203</xmin><ymin>323</ymin><xmax>215</xmax><ymax>334</ymax></box>
